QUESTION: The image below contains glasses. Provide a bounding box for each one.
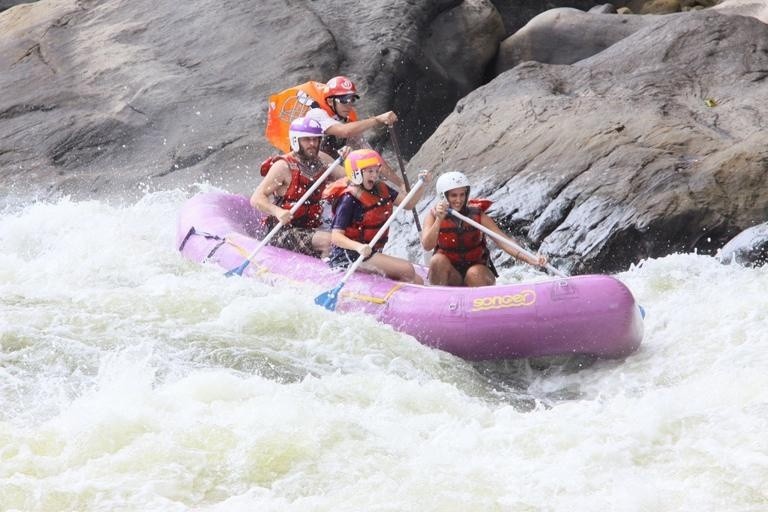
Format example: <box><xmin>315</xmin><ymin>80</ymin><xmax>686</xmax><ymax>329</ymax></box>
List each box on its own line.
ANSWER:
<box><xmin>331</xmin><ymin>98</ymin><xmax>356</xmax><ymax>105</ymax></box>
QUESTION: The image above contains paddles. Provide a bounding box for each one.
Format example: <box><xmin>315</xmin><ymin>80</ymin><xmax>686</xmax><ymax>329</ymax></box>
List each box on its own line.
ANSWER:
<box><xmin>224</xmin><ymin>158</ymin><xmax>339</xmax><ymax>276</ymax></box>
<box><xmin>314</xmin><ymin>176</ymin><xmax>423</xmax><ymax>311</ymax></box>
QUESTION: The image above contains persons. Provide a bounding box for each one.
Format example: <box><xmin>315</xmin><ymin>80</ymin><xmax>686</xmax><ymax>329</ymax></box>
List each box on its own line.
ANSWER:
<box><xmin>249</xmin><ymin>76</ymin><xmax>547</xmax><ymax>286</ymax></box>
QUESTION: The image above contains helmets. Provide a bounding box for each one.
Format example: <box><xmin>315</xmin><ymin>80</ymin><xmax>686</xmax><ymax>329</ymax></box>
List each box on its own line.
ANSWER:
<box><xmin>436</xmin><ymin>171</ymin><xmax>471</xmax><ymax>207</ymax></box>
<box><xmin>344</xmin><ymin>148</ymin><xmax>385</xmax><ymax>185</ymax></box>
<box><xmin>288</xmin><ymin>117</ymin><xmax>325</xmax><ymax>154</ymax></box>
<box><xmin>323</xmin><ymin>75</ymin><xmax>360</xmax><ymax>102</ymax></box>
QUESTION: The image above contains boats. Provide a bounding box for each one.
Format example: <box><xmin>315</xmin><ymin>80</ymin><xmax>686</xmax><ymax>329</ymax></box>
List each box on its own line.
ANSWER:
<box><xmin>176</xmin><ymin>190</ymin><xmax>645</xmax><ymax>363</ymax></box>
